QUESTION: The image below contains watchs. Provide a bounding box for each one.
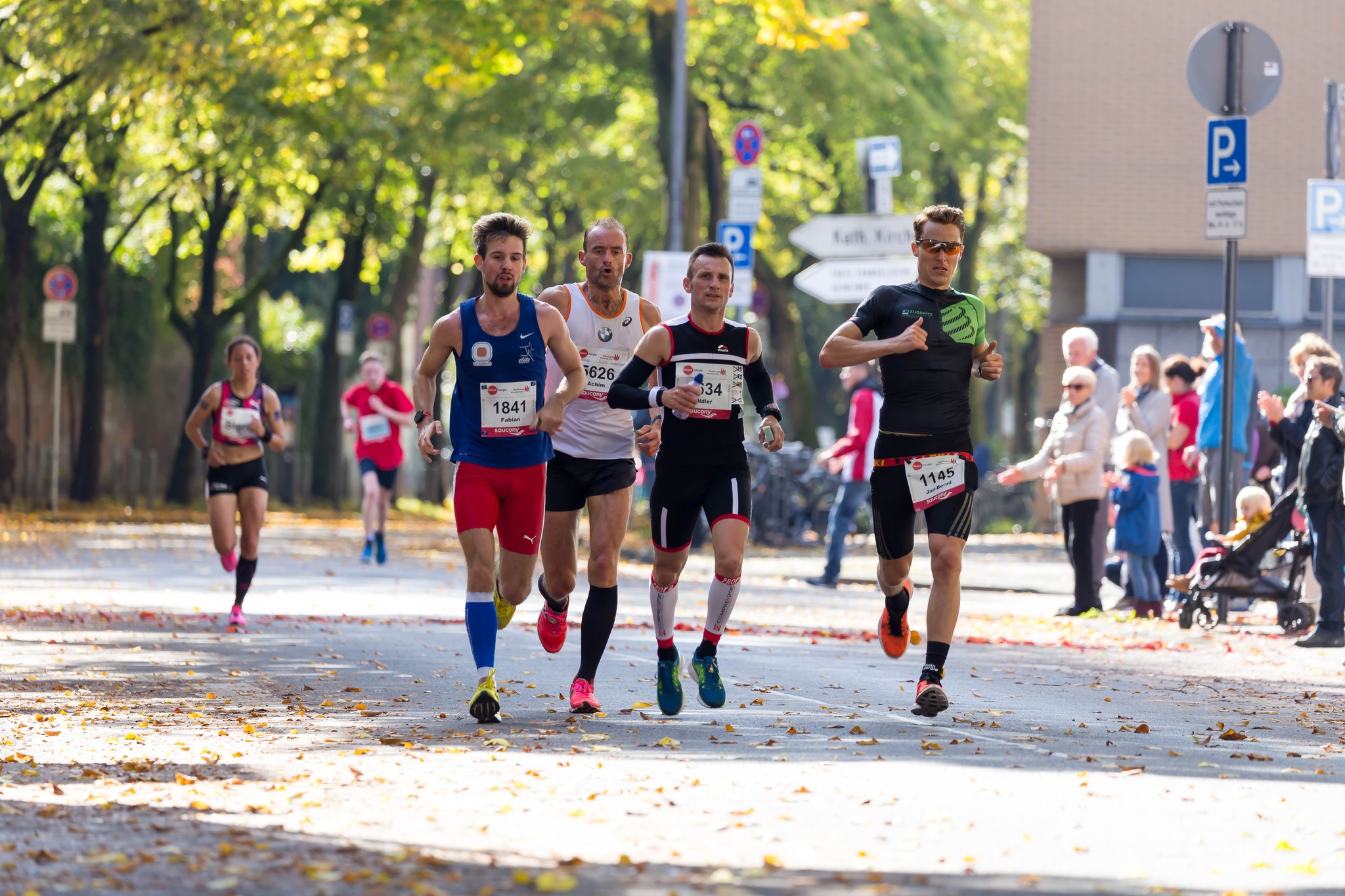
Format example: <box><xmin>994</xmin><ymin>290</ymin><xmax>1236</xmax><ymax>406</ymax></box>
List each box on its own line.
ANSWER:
<box><xmin>758</xmin><ymin>402</ymin><xmax>785</xmax><ymax>424</ymax></box>
<box><xmin>413</xmin><ymin>410</ymin><xmax>433</xmax><ymax>428</ymax></box>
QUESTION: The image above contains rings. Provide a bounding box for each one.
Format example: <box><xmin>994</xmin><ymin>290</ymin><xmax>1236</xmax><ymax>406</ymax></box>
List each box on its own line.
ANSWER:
<box><xmin>781</xmin><ymin>442</ymin><xmax>784</xmax><ymax>445</ymax></box>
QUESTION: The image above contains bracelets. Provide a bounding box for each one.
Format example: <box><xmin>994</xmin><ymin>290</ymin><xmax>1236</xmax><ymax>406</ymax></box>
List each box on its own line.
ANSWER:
<box><xmin>978</xmin><ymin>362</ymin><xmax>988</xmax><ymax>381</ymax></box>
<box><xmin>201</xmin><ymin>446</ymin><xmax>209</xmax><ymax>460</ymax></box>
<box><xmin>648</xmin><ymin>414</ymin><xmax>663</xmax><ymax>425</ymax></box>
<box><xmin>649</xmin><ymin>385</ymin><xmax>667</xmax><ymax>408</ymax></box>
<box><xmin>261</xmin><ymin>427</ymin><xmax>273</xmax><ymax>445</ymax></box>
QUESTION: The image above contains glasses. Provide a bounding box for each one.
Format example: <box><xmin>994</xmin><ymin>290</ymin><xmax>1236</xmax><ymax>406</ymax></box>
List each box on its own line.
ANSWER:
<box><xmin>916</xmin><ymin>238</ymin><xmax>964</xmax><ymax>255</ymax></box>
<box><xmin>1063</xmin><ymin>383</ymin><xmax>1088</xmax><ymax>391</ymax></box>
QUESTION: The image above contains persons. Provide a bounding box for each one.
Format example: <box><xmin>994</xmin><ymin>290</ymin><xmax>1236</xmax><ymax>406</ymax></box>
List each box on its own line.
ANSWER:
<box><xmin>607</xmin><ymin>242</ymin><xmax>784</xmax><ymax>716</ymax></box>
<box><xmin>1105</xmin><ymin>429</ymin><xmax>1167</xmax><ymax>623</ymax></box>
<box><xmin>184</xmin><ymin>335</ymin><xmax>285</xmax><ymax>631</ymax></box>
<box><xmin>967</xmin><ymin>313</ymin><xmax>1345</xmax><ymax>648</ymax></box>
<box><xmin>820</xmin><ymin>204</ymin><xmax>1004</xmax><ymax>717</ymax></box>
<box><xmin>412</xmin><ymin>213</ymin><xmax>587</xmax><ymax>724</ymax></box>
<box><xmin>340</xmin><ymin>348</ymin><xmax>414</xmax><ymax>564</ymax></box>
<box><xmin>1195</xmin><ymin>313</ymin><xmax>1255</xmax><ymax>611</ymax></box>
<box><xmin>995</xmin><ymin>364</ymin><xmax>1110</xmax><ymax>617</ymax></box>
<box><xmin>536</xmin><ymin>218</ymin><xmax>666</xmax><ymax>714</ymax></box>
<box><xmin>805</xmin><ymin>358</ymin><xmax>885</xmax><ymax>589</ymax></box>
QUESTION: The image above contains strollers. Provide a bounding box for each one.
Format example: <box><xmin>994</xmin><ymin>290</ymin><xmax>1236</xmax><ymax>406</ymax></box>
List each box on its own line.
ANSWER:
<box><xmin>1178</xmin><ymin>478</ymin><xmax>1317</xmax><ymax>632</ymax></box>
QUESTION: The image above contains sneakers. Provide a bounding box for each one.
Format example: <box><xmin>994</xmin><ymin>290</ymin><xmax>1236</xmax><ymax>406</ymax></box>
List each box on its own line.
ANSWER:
<box><xmin>493</xmin><ymin>559</ymin><xmax>516</xmax><ymax>629</ymax></box>
<box><xmin>687</xmin><ymin>646</ymin><xmax>726</xmax><ymax>708</ymax></box>
<box><xmin>878</xmin><ymin>577</ymin><xmax>914</xmax><ymax>658</ymax></box>
<box><xmin>230</xmin><ymin>605</ymin><xmax>246</xmax><ymax>626</ymax></box>
<box><xmin>570</xmin><ymin>678</ymin><xmax>601</xmax><ymax>713</ymax></box>
<box><xmin>219</xmin><ymin>549</ymin><xmax>237</xmax><ymax>573</ymax></box>
<box><xmin>537</xmin><ymin>594</ymin><xmax>569</xmax><ymax>654</ymax></box>
<box><xmin>469</xmin><ymin>667</ymin><xmax>502</xmax><ymax>724</ymax></box>
<box><xmin>359</xmin><ymin>547</ymin><xmax>372</xmax><ymax>563</ymax></box>
<box><xmin>654</xmin><ymin>645</ymin><xmax>685</xmax><ymax>715</ymax></box>
<box><xmin>374</xmin><ymin>532</ymin><xmax>387</xmax><ymax>564</ymax></box>
<box><xmin>910</xmin><ymin>679</ymin><xmax>949</xmax><ymax>717</ymax></box>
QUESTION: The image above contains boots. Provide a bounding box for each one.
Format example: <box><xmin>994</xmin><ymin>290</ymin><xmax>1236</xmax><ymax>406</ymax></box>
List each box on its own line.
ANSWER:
<box><xmin>1149</xmin><ymin>599</ymin><xmax>1163</xmax><ymax>618</ymax></box>
<box><xmin>1134</xmin><ymin>599</ymin><xmax>1150</xmax><ymax>620</ymax></box>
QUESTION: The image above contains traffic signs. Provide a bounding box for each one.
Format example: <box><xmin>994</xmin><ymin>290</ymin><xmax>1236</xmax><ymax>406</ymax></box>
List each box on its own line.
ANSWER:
<box><xmin>793</xmin><ymin>253</ymin><xmax>926</xmax><ymax>303</ymax></box>
<box><xmin>789</xmin><ymin>210</ymin><xmax>924</xmax><ymax>260</ymax></box>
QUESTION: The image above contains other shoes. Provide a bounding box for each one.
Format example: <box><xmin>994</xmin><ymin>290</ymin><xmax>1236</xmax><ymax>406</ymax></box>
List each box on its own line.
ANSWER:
<box><xmin>1294</xmin><ymin>629</ymin><xmax>1345</xmax><ymax>647</ymax></box>
<box><xmin>805</xmin><ymin>576</ymin><xmax>838</xmax><ymax>589</ymax></box>
<box><xmin>1054</xmin><ymin>606</ymin><xmax>1078</xmax><ymax>617</ymax></box>
<box><xmin>1164</xmin><ymin>573</ymin><xmax>1195</xmax><ymax>595</ymax></box>
<box><xmin>1114</xmin><ymin>596</ymin><xmax>1133</xmax><ymax>608</ymax></box>
